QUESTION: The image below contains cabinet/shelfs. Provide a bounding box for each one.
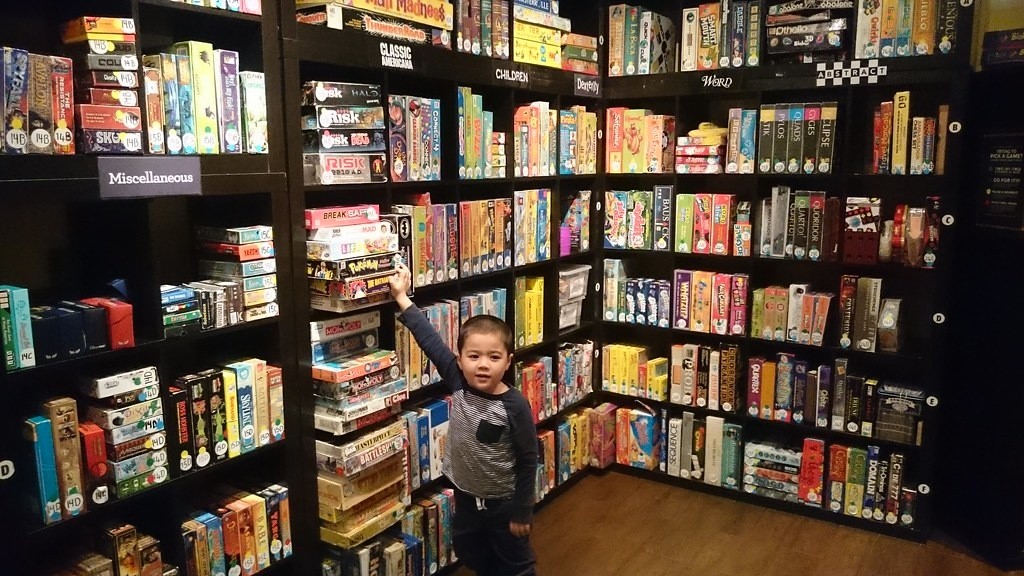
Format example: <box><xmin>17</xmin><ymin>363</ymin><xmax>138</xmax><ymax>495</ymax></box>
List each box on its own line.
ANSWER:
<box><xmin>0</xmin><ymin>0</ymin><xmax>975</xmax><ymax>576</ymax></box>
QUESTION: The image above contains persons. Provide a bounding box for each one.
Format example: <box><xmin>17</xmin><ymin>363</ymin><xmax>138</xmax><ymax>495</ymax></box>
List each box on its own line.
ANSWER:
<box><xmin>388</xmin><ymin>261</ymin><xmax>538</xmax><ymax>575</ymax></box>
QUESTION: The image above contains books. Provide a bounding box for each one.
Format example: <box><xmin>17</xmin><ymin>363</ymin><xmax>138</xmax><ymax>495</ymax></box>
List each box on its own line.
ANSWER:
<box><xmin>297</xmin><ymin>0</ymin><xmax>960</xmax><ymax>576</ymax></box>
<box><xmin>981</xmin><ymin>28</ymin><xmax>1024</xmax><ymax>68</ymax></box>
<box><xmin>975</xmin><ymin>133</ymin><xmax>1024</xmax><ymax>234</ymax></box>
<box><xmin>1</xmin><ymin>0</ymin><xmax>295</xmax><ymax>576</ymax></box>
